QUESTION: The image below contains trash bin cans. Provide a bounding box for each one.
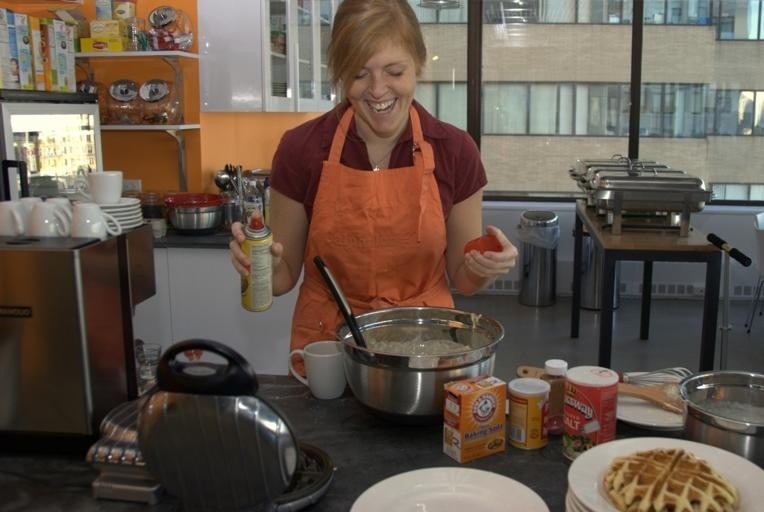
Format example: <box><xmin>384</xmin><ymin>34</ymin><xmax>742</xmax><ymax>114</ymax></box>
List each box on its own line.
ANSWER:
<box><xmin>572</xmin><ymin>224</ymin><xmax>620</xmax><ymax>310</ymax></box>
<box><xmin>518</xmin><ymin>211</ymin><xmax>559</xmax><ymax>306</ymax></box>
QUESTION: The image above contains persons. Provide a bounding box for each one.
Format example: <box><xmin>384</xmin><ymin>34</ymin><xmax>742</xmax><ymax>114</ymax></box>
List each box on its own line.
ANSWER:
<box><xmin>230</xmin><ymin>0</ymin><xmax>518</xmax><ymax>378</ymax></box>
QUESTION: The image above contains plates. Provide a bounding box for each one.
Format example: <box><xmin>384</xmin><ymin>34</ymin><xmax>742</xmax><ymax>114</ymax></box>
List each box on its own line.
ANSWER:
<box><xmin>251</xmin><ymin>168</ymin><xmax>270</xmax><ymax>176</ymax></box>
<box><xmin>271</xmin><ymin>80</ymin><xmax>285</xmax><ymax>96</ymax></box>
<box><xmin>564</xmin><ymin>436</ymin><xmax>764</xmax><ymax>510</ymax></box>
<box><xmin>348</xmin><ymin>466</ymin><xmax>550</xmax><ymax>511</ymax></box>
<box><xmin>74</xmin><ymin>197</ymin><xmax>144</xmax><ymax>233</ymax></box>
<box><xmin>613</xmin><ymin>370</ymin><xmax>684</xmax><ymax>430</ymax></box>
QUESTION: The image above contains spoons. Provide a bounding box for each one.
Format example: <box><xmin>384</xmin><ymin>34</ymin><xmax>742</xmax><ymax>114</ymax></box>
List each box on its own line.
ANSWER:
<box><xmin>214</xmin><ymin>165</ymin><xmax>240</xmax><ymax>196</ymax></box>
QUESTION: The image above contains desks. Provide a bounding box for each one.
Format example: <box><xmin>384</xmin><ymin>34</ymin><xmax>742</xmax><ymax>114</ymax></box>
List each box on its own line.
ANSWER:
<box><xmin>570</xmin><ymin>197</ymin><xmax>723</xmax><ymax>371</ymax></box>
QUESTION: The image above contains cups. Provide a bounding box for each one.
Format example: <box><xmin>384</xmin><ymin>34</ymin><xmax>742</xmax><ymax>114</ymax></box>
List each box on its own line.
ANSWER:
<box><xmin>74</xmin><ymin>165</ymin><xmax>123</xmax><ymax>201</ymax></box>
<box><xmin>287</xmin><ymin>340</ymin><xmax>345</xmax><ymax>399</ymax></box>
<box><xmin>0</xmin><ymin>197</ymin><xmax>121</xmax><ymax>241</ymax></box>
<box><xmin>223</xmin><ymin>203</ymin><xmax>241</xmax><ymax>230</ymax></box>
<box><xmin>135</xmin><ymin>344</ymin><xmax>161</xmax><ymax>379</ymax></box>
<box><xmin>463</xmin><ymin>236</ymin><xmax>502</xmax><ymax>255</ymax></box>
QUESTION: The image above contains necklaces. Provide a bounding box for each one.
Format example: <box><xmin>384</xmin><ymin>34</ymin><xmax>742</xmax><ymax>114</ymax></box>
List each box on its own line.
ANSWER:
<box><xmin>370</xmin><ymin>152</ymin><xmax>390</xmax><ymax>172</ymax></box>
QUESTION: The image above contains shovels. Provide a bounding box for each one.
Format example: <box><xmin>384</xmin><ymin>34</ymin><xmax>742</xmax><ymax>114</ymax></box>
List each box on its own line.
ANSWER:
<box><xmin>516</xmin><ymin>366</ymin><xmax>684</xmax><ymax>415</ymax></box>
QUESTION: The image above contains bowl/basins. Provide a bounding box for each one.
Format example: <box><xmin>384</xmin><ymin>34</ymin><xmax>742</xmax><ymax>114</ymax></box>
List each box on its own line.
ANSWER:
<box><xmin>169</xmin><ymin>207</ymin><xmax>222</xmax><ymax>234</ymax></box>
<box><xmin>336</xmin><ymin>305</ymin><xmax>506</xmax><ymax>420</ymax></box>
<box><xmin>679</xmin><ymin>369</ymin><xmax>764</xmax><ymax>466</ymax></box>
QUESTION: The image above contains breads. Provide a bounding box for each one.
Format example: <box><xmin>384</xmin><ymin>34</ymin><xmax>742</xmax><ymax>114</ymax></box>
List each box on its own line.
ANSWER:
<box><xmin>602</xmin><ymin>448</ymin><xmax>739</xmax><ymax>512</ymax></box>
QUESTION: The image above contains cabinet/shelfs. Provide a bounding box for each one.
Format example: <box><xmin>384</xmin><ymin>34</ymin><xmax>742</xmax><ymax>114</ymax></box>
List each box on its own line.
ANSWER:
<box><xmin>0</xmin><ymin>88</ymin><xmax>104</xmax><ymax>198</ymax></box>
<box><xmin>75</xmin><ymin>49</ymin><xmax>201</xmax><ymax>191</ymax></box>
<box><xmin>196</xmin><ymin>0</ymin><xmax>317</xmax><ymax>114</ymax></box>
<box><xmin>317</xmin><ymin>1</ymin><xmax>343</xmax><ymax>114</ymax></box>
<box><xmin>131</xmin><ymin>247</ymin><xmax>304</xmax><ymax>376</ymax></box>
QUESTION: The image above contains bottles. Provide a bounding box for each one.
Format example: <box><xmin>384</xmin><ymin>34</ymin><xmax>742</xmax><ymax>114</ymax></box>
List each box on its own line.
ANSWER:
<box><xmin>507</xmin><ymin>378</ymin><xmax>549</xmax><ymax>450</ymax></box>
<box><xmin>122</xmin><ymin>190</ymin><xmax>174</xmax><ymax>217</ymax></box>
<box><xmin>121</xmin><ymin>16</ymin><xmax>146</xmax><ymax>50</ymax></box>
<box><xmin>539</xmin><ymin>357</ymin><xmax>567</xmax><ymax>418</ymax></box>
<box><xmin>243</xmin><ymin>181</ymin><xmax>263</xmax><ymax>221</ymax></box>
<box><xmin>27</xmin><ymin>131</ymin><xmax>41</xmax><ymax>173</ymax></box>
<box><xmin>145</xmin><ymin>5</ymin><xmax>193</xmax><ymax>52</ymax></box>
<box><xmin>78</xmin><ymin>80</ymin><xmax>183</xmax><ymax>125</ymax></box>
<box><xmin>561</xmin><ymin>365</ymin><xmax>618</xmax><ymax>461</ymax></box>
<box><xmin>12</xmin><ymin>132</ymin><xmax>29</xmax><ymax>173</ymax></box>
